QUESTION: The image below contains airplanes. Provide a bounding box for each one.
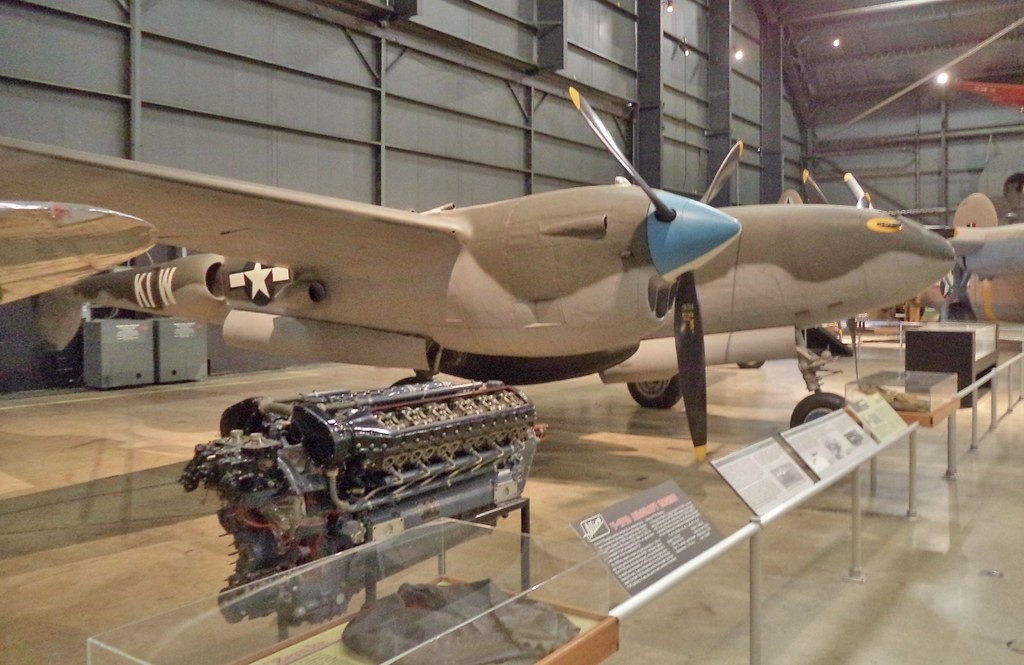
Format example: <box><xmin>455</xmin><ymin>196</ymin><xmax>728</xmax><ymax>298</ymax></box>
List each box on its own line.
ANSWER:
<box><xmin>0</xmin><ymin>85</ymin><xmax>960</xmax><ymax>467</ymax></box>
<box><xmin>771</xmin><ymin>167</ymin><xmax>1024</xmax><ymax>340</ymax></box>
